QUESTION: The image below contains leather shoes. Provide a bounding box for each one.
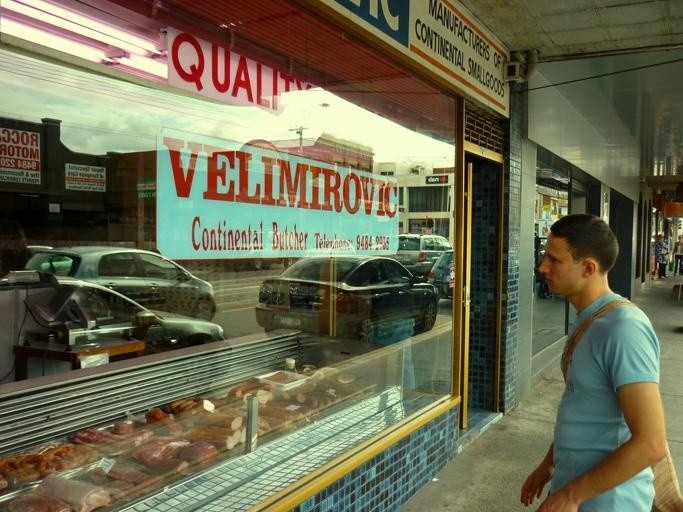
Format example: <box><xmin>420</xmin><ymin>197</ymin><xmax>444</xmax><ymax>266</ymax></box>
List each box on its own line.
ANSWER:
<box><xmin>661</xmin><ymin>276</ymin><xmax>665</xmax><ymax>278</ymax></box>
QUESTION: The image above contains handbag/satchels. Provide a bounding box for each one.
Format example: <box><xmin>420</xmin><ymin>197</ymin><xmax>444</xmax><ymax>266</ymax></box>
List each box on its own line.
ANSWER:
<box><xmin>663</xmin><ymin>255</ymin><xmax>669</xmax><ymax>261</ymax></box>
<box><xmin>560</xmin><ymin>298</ymin><xmax>681</xmax><ymax>508</ymax></box>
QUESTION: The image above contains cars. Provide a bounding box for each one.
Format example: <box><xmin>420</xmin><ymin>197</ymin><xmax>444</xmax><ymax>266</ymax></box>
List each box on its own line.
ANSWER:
<box><xmin>0</xmin><ymin>277</ymin><xmax>232</xmax><ymax>398</ymax></box>
<box><xmin>255</xmin><ymin>256</ymin><xmax>439</xmax><ymax>355</ymax></box>
<box><xmin>427</xmin><ymin>250</ymin><xmax>455</xmax><ymax>301</ymax></box>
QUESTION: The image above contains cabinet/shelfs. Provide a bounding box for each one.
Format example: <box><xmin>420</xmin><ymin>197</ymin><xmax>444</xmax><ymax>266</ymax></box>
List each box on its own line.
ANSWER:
<box><xmin>0</xmin><ymin>328</ymin><xmax>405</xmax><ymax>512</ymax></box>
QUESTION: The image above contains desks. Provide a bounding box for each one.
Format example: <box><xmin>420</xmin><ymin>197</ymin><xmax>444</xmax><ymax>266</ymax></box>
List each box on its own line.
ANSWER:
<box><xmin>13</xmin><ymin>336</ymin><xmax>145</xmax><ymax>381</ymax></box>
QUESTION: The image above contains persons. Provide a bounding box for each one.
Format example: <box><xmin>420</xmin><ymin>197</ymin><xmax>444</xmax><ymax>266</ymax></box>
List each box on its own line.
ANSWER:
<box><xmin>656</xmin><ymin>234</ymin><xmax>668</xmax><ymax>278</ymax></box>
<box><xmin>521</xmin><ymin>214</ymin><xmax>667</xmax><ymax>512</ymax></box>
<box><xmin>672</xmin><ymin>235</ymin><xmax>683</xmax><ymax>275</ymax></box>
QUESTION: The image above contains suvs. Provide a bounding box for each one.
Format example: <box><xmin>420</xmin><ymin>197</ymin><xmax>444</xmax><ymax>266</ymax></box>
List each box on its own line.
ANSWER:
<box><xmin>24</xmin><ymin>245</ymin><xmax>216</xmax><ymax>322</ymax></box>
<box><xmin>394</xmin><ymin>234</ymin><xmax>453</xmax><ymax>273</ymax></box>
